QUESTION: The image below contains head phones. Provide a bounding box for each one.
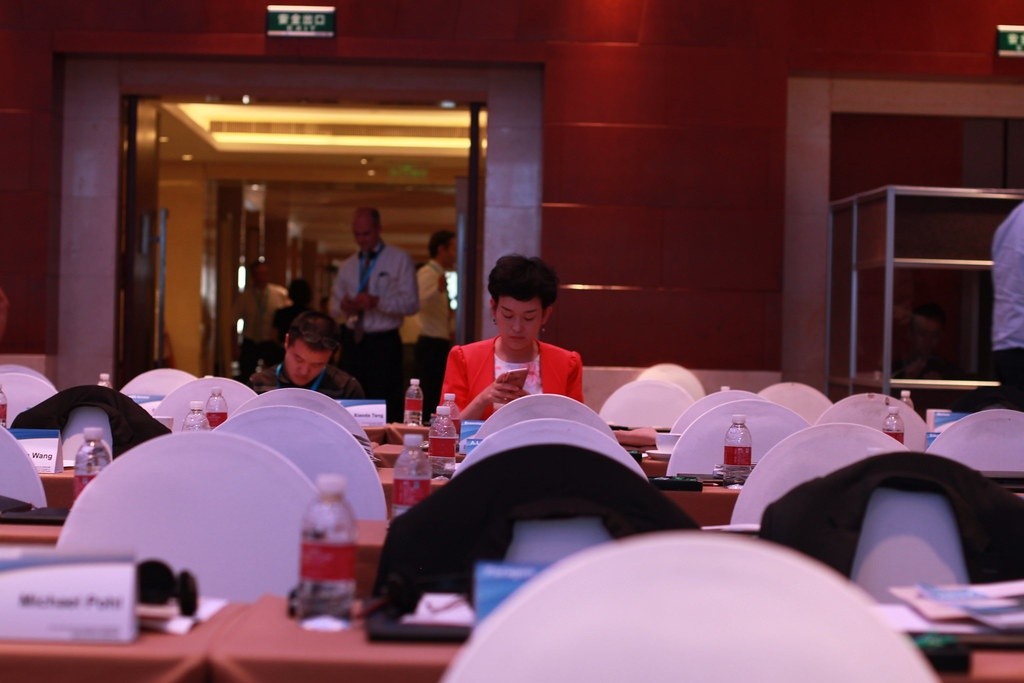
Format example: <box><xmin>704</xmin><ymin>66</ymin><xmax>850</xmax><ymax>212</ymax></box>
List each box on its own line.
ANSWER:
<box><xmin>136</xmin><ymin>560</ymin><xmax>198</xmax><ymax>618</ymax></box>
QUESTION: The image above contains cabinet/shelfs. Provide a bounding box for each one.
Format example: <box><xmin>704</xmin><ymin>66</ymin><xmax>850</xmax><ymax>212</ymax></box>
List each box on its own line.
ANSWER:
<box><xmin>827</xmin><ymin>182</ymin><xmax>1024</xmax><ymax>394</ymax></box>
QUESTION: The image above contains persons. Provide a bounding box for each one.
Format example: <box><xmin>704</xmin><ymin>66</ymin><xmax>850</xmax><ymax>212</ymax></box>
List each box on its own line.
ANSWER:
<box><xmin>328</xmin><ymin>207</ymin><xmax>420</xmax><ymax>422</ymax></box>
<box><xmin>245</xmin><ymin>308</ymin><xmax>364</xmax><ymax>401</ymax></box>
<box><xmin>993</xmin><ymin>204</ymin><xmax>1024</xmax><ymax>412</ymax></box>
<box><xmin>406</xmin><ymin>230</ymin><xmax>457</xmax><ymax>420</ymax></box>
<box><xmin>270</xmin><ymin>279</ymin><xmax>316</xmax><ymax>364</ymax></box>
<box><xmin>437</xmin><ymin>254</ymin><xmax>583</xmax><ymax>432</ymax></box>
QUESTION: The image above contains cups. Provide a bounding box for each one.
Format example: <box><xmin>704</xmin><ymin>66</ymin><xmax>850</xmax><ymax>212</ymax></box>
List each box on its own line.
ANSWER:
<box><xmin>655</xmin><ymin>433</ymin><xmax>681</xmax><ymax>452</ymax></box>
<box><xmin>152</xmin><ymin>415</ymin><xmax>174</xmax><ymax>429</ymax></box>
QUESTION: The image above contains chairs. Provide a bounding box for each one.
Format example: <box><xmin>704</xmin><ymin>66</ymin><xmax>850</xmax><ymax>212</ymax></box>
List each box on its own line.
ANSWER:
<box><xmin>211</xmin><ymin>404</ymin><xmax>389</xmax><ymax>521</ymax></box>
<box><xmin>372</xmin><ymin>442</ymin><xmax>698</xmax><ymax>609</ymax></box>
<box><xmin>670</xmin><ymin>390</ymin><xmax>772</xmax><ymax>433</ymax></box>
<box><xmin>435</xmin><ymin>530</ymin><xmax>944</xmax><ymax>683</ymax></box>
<box><xmin>664</xmin><ymin>399</ymin><xmax>813</xmax><ymax>477</ymax></box>
<box><xmin>728</xmin><ymin>422</ymin><xmax>912</xmax><ymax>524</ymax></box>
<box><xmin>474</xmin><ymin>393</ymin><xmax>620</xmax><ymax>444</ymax></box>
<box><xmin>0</xmin><ymin>372</ymin><xmax>59</xmax><ymax>431</ymax></box>
<box><xmin>639</xmin><ymin>363</ymin><xmax>708</xmax><ymax>401</ymax></box>
<box><xmin>757</xmin><ymin>381</ymin><xmax>833</xmax><ymax>424</ymax></box>
<box><xmin>0</xmin><ymin>425</ymin><xmax>48</xmax><ymax>511</ymax></box>
<box><xmin>0</xmin><ymin>363</ymin><xmax>55</xmax><ymax>386</ymax></box>
<box><xmin>597</xmin><ymin>380</ymin><xmax>695</xmax><ymax>430</ymax></box>
<box><xmin>119</xmin><ymin>367</ymin><xmax>198</xmax><ymax>410</ymax></box>
<box><xmin>814</xmin><ymin>393</ymin><xmax>928</xmax><ymax>453</ymax></box>
<box><xmin>154</xmin><ymin>376</ymin><xmax>259</xmax><ymax>431</ymax></box>
<box><xmin>54</xmin><ymin>430</ymin><xmax>322</xmax><ymax>602</ymax></box>
<box><xmin>227</xmin><ymin>388</ymin><xmax>378</xmax><ymax>465</ymax></box>
<box><xmin>927</xmin><ymin>407</ymin><xmax>1024</xmax><ymax>471</ymax></box>
<box><xmin>758</xmin><ymin>450</ymin><xmax>1024</xmax><ymax>606</ymax></box>
<box><xmin>449</xmin><ymin>417</ymin><xmax>650</xmax><ymax>483</ymax></box>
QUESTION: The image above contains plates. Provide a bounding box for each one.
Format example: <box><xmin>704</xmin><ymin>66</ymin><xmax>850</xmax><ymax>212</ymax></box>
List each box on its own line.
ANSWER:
<box><xmin>646</xmin><ymin>449</ymin><xmax>672</xmax><ymax>460</ymax></box>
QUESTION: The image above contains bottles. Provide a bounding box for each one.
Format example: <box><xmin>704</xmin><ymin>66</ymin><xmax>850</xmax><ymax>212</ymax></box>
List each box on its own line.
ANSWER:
<box><xmin>428</xmin><ymin>406</ymin><xmax>456</xmax><ymax>479</ymax></box>
<box><xmin>97</xmin><ymin>373</ymin><xmax>113</xmax><ymax>388</ymax></box>
<box><xmin>75</xmin><ymin>427</ymin><xmax>113</xmax><ymax>501</ymax></box>
<box><xmin>404</xmin><ymin>378</ymin><xmax>423</xmax><ymax>427</ymax></box>
<box><xmin>255</xmin><ymin>358</ymin><xmax>264</xmax><ymax>375</ymax></box>
<box><xmin>900</xmin><ymin>390</ymin><xmax>913</xmax><ymax>409</ymax></box>
<box><xmin>205</xmin><ymin>387</ymin><xmax>227</xmax><ymax>430</ymax></box>
<box><xmin>0</xmin><ymin>384</ymin><xmax>7</xmax><ymax>429</ymax></box>
<box><xmin>392</xmin><ymin>434</ymin><xmax>432</xmax><ymax>519</ymax></box>
<box><xmin>723</xmin><ymin>414</ymin><xmax>752</xmax><ymax>490</ymax></box>
<box><xmin>882</xmin><ymin>406</ymin><xmax>904</xmax><ymax>444</ymax></box>
<box><xmin>181</xmin><ymin>401</ymin><xmax>210</xmax><ymax>431</ymax></box>
<box><xmin>443</xmin><ymin>393</ymin><xmax>461</xmax><ymax>435</ymax></box>
<box><xmin>296</xmin><ymin>473</ymin><xmax>358</xmax><ymax>633</ymax></box>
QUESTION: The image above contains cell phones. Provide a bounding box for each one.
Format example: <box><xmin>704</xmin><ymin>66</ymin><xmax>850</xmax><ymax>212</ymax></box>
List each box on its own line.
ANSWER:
<box><xmin>500</xmin><ymin>367</ymin><xmax>529</xmax><ymax>401</ymax></box>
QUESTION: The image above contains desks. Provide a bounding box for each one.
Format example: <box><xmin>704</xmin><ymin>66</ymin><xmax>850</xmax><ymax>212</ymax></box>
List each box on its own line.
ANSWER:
<box><xmin>0</xmin><ymin>424</ymin><xmax>1024</xmax><ymax>683</ymax></box>
